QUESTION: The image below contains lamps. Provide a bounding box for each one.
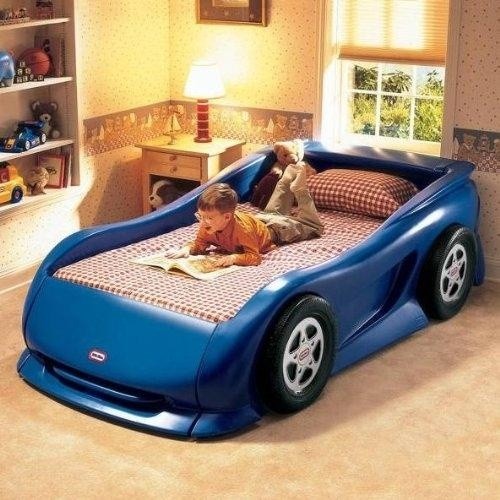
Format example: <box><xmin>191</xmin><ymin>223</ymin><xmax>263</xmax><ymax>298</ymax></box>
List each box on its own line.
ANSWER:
<box><xmin>184</xmin><ymin>62</ymin><xmax>225</xmax><ymax>143</ymax></box>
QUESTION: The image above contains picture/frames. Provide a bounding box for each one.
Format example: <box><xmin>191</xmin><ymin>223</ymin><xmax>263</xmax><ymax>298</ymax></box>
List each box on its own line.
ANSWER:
<box><xmin>197</xmin><ymin>0</ymin><xmax>265</xmax><ymax>26</ymax></box>
<box><xmin>37</xmin><ymin>153</ymin><xmax>65</xmax><ymax>190</ymax></box>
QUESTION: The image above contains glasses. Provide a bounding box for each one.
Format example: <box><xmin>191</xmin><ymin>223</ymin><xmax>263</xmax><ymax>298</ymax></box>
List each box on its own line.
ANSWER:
<box><xmin>194</xmin><ymin>211</ymin><xmax>226</xmax><ymax>220</ymax></box>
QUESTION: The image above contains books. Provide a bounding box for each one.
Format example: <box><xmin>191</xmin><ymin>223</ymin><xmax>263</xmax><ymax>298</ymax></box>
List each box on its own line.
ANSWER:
<box><xmin>132</xmin><ymin>248</ymin><xmax>240</xmax><ymax>281</ymax></box>
<box><xmin>35</xmin><ymin>36</ymin><xmax>65</xmax><ymax>77</ymax></box>
<box><xmin>36</xmin><ymin>145</ymin><xmax>73</xmax><ymax>188</ymax></box>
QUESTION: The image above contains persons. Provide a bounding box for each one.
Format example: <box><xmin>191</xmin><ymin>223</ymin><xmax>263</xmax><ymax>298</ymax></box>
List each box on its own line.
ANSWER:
<box><xmin>165</xmin><ymin>165</ymin><xmax>324</xmax><ymax>268</ymax></box>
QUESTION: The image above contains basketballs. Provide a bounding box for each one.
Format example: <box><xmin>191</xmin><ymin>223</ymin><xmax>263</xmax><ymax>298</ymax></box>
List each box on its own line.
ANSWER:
<box><xmin>19</xmin><ymin>49</ymin><xmax>50</xmax><ymax>77</ymax></box>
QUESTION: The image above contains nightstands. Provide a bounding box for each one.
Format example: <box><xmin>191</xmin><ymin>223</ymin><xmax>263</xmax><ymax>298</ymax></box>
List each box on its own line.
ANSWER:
<box><xmin>134</xmin><ymin>134</ymin><xmax>247</xmax><ymax>218</ymax></box>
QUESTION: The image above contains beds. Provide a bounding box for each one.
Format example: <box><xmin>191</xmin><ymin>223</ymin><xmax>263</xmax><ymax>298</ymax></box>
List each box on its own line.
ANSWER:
<box><xmin>17</xmin><ymin>138</ymin><xmax>485</xmax><ymax>441</ymax></box>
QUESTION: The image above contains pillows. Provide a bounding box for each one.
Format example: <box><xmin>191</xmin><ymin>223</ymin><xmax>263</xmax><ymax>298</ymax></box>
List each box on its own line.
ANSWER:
<box><xmin>297</xmin><ymin>168</ymin><xmax>417</xmax><ymax>219</ymax></box>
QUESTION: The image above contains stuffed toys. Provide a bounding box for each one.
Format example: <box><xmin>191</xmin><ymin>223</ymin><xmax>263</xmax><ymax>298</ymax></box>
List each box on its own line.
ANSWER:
<box><xmin>149</xmin><ymin>180</ymin><xmax>185</xmax><ymax>210</ymax></box>
<box><xmin>271</xmin><ymin>139</ymin><xmax>316</xmax><ymax>186</ymax></box>
<box><xmin>31</xmin><ymin>100</ymin><xmax>60</xmax><ymax>141</ymax></box>
<box><xmin>25</xmin><ymin>167</ymin><xmax>49</xmax><ymax>195</ymax></box>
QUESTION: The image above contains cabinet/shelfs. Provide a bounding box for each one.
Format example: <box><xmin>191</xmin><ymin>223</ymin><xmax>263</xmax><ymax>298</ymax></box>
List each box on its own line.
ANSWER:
<box><xmin>0</xmin><ymin>0</ymin><xmax>82</xmax><ymax>220</ymax></box>
